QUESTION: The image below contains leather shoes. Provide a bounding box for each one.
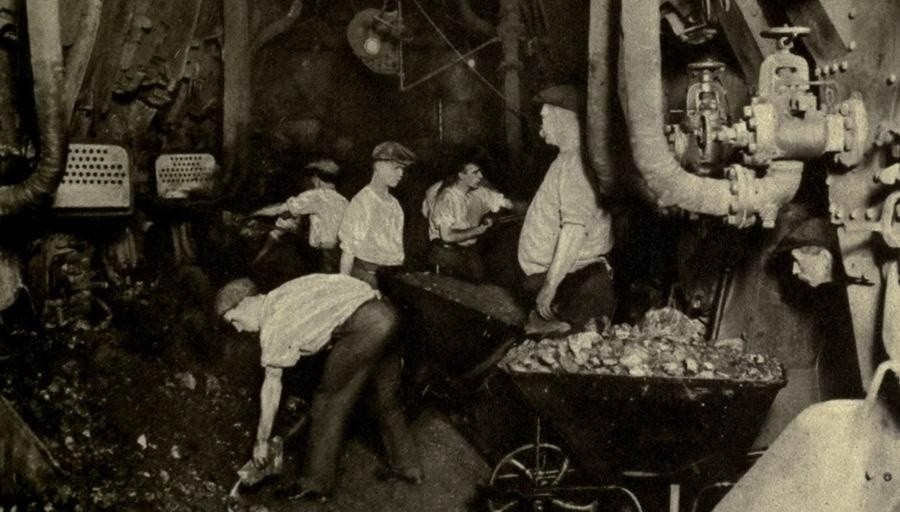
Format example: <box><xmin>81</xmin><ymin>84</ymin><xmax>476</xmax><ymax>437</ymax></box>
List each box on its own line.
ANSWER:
<box><xmin>377</xmin><ymin>464</ymin><xmax>426</xmax><ymax>484</ymax></box>
<box><xmin>276</xmin><ymin>484</ymin><xmax>333</xmax><ymax>504</ymax></box>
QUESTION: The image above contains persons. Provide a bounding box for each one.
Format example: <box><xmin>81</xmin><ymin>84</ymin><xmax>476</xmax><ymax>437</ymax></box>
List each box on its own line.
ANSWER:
<box><xmin>210</xmin><ymin>269</ymin><xmax>427</xmax><ymax>505</ymax></box>
<box><xmin>516</xmin><ymin>81</ymin><xmax>621</xmax><ymax>342</ymax></box>
<box><xmin>235</xmin><ymin>158</ymin><xmax>350</xmax><ymax>273</ymax></box>
<box><xmin>338</xmin><ymin>140</ymin><xmax>418</xmax><ymax>287</ymax></box>
<box><xmin>422</xmin><ymin>147</ymin><xmax>528</xmax><ymax>287</ymax></box>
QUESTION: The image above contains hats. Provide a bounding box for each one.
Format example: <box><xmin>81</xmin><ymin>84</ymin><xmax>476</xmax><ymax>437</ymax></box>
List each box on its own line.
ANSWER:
<box><xmin>307</xmin><ymin>161</ymin><xmax>338</xmax><ymax>176</ymax></box>
<box><xmin>374</xmin><ymin>142</ymin><xmax>415</xmax><ymax>164</ymax></box>
<box><xmin>533</xmin><ymin>83</ymin><xmax>581</xmax><ymax>112</ymax></box>
<box><xmin>215</xmin><ymin>279</ymin><xmax>258</xmax><ymax>317</ymax></box>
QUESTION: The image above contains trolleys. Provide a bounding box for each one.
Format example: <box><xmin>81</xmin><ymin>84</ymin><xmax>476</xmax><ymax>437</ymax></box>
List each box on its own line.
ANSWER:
<box><xmin>491</xmin><ymin>324</ymin><xmax>784</xmax><ymax>512</ymax></box>
